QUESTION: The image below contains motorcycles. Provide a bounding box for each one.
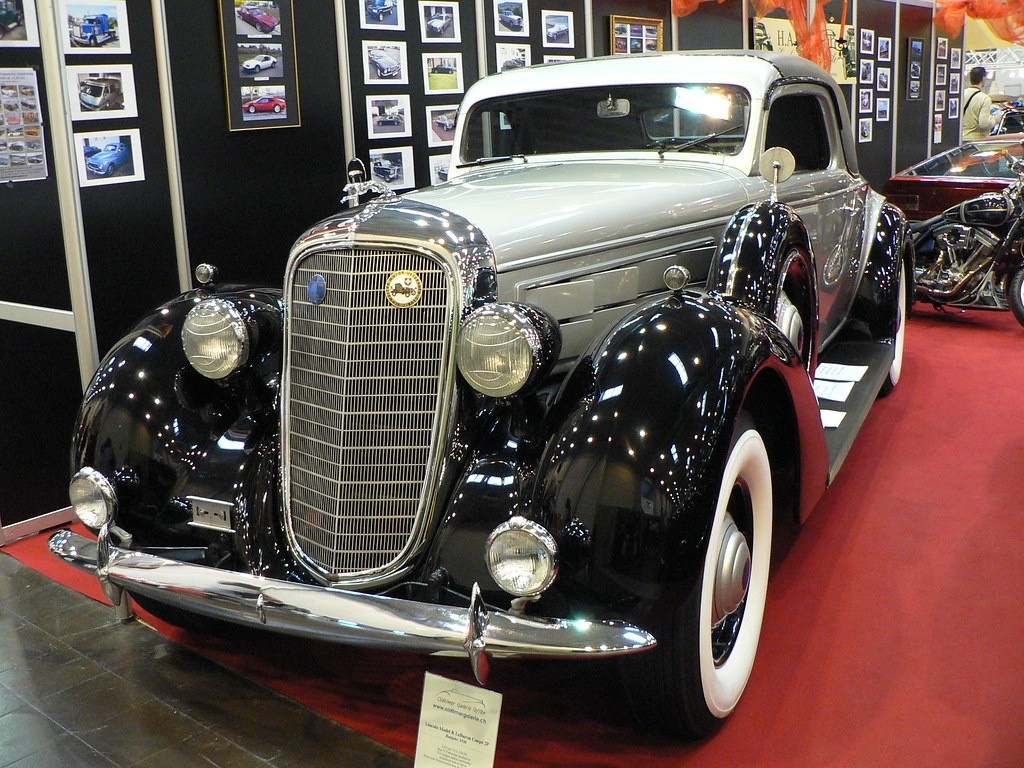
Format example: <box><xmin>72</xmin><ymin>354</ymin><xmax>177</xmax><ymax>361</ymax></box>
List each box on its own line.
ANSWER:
<box><xmin>906</xmin><ymin>148</ymin><xmax>1024</xmax><ymax>327</ymax></box>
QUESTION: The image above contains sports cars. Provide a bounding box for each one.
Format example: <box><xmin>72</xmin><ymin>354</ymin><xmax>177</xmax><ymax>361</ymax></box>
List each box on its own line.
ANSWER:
<box><xmin>238</xmin><ymin>6</ymin><xmax>286</xmax><ymax>114</ymax></box>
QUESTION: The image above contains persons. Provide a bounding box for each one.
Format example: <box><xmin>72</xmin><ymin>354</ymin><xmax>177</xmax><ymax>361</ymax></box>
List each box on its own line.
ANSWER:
<box><xmin>961</xmin><ymin>67</ymin><xmax>1003</xmax><ymax>144</ymax></box>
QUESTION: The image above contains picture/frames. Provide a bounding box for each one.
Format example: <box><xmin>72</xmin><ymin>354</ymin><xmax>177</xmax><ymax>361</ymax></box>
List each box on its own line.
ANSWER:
<box><xmin>65</xmin><ymin>64</ymin><xmax>139</xmax><ymax>122</ymax></box>
<box><xmin>492</xmin><ymin>0</ymin><xmax>575</xmax><ymax>130</ymax></box>
<box><xmin>366</xmin><ymin>94</ymin><xmax>412</xmax><ymax>139</ymax></box>
<box><xmin>0</xmin><ymin>0</ymin><xmax>40</xmax><ymax>48</ymax></box>
<box><xmin>368</xmin><ymin>146</ymin><xmax>415</xmax><ymax>194</ymax></box>
<box><xmin>422</xmin><ymin>52</ymin><xmax>465</xmax><ymax>95</ymax></box>
<box><xmin>610</xmin><ymin>14</ymin><xmax>663</xmax><ymax>55</ymax></box>
<box><xmin>358</xmin><ymin>0</ymin><xmax>405</xmax><ymax>30</ymax></box>
<box><xmin>428</xmin><ymin>154</ymin><xmax>451</xmax><ymax>185</ymax></box>
<box><xmin>418</xmin><ymin>0</ymin><xmax>461</xmax><ymax>43</ymax></box>
<box><xmin>425</xmin><ymin>103</ymin><xmax>458</xmax><ymax>148</ymax></box>
<box><xmin>934</xmin><ymin>36</ymin><xmax>960</xmax><ymax>118</ymax></box>
<box><xmin>362</xmin><ymin>40</ymin><xmax>409</xmax><ymax>85</ymax></box>
<box><xmin>59</xmin><ymin>0</ymin><xmax>131</xmax><ymax>54</ymax></box>
<box><xmin>858</xmin><ymin>29</ymin><xmax>891</xmax><ymax>143</ymax></box>
<box><xmin>73</xmin><ymin>127</ymin><xmax>145</xmax><ymax>188</ymax></box>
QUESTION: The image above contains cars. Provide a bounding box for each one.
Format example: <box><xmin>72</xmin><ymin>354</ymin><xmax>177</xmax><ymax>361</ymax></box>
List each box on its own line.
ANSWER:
<box><xmin>366</xmin><ymin>0</ymin><xmax>403</xmax><ymax>182</ymax></box>
<box><xmin>70</xmin><ymin>13</ymin><xmax>129</xmax><ymax>176</ymax></box>
<box><xmin>499</xmin><ymin>11</ymin><xmax>522</xmax><ymax>28</ymax></box>
<box><xmin>547</xmin><ymin>24</ymin><xmax>568</xmax><ymax>39</ymax></box>
<box><xmin>979</xmin><ymin>101</ymin><xmax>1024</xmax><ymax>141</ymax></box>
<box><xmin>48</xmin><ymin>50</ymin><xmax>916</xmax><ymax>737</ymax></box>
<box><xmin>432</xmin><ymin>65</ymin><xmax>457</xmax><ymax>74</ymax></box>
<box><xmin>435</xmin><ymin>116</ymin><xmax>454</xmax><ymax>130</ymax></box>
<box><xmin>427</xmin><ymin>14</ymin><xmax>452</xmax><ymax>35</ymax></box>
<box><xmin>615</xmin><ymin>23</ymin><xmax>657</xmax><ymax>54</ymax></box>
